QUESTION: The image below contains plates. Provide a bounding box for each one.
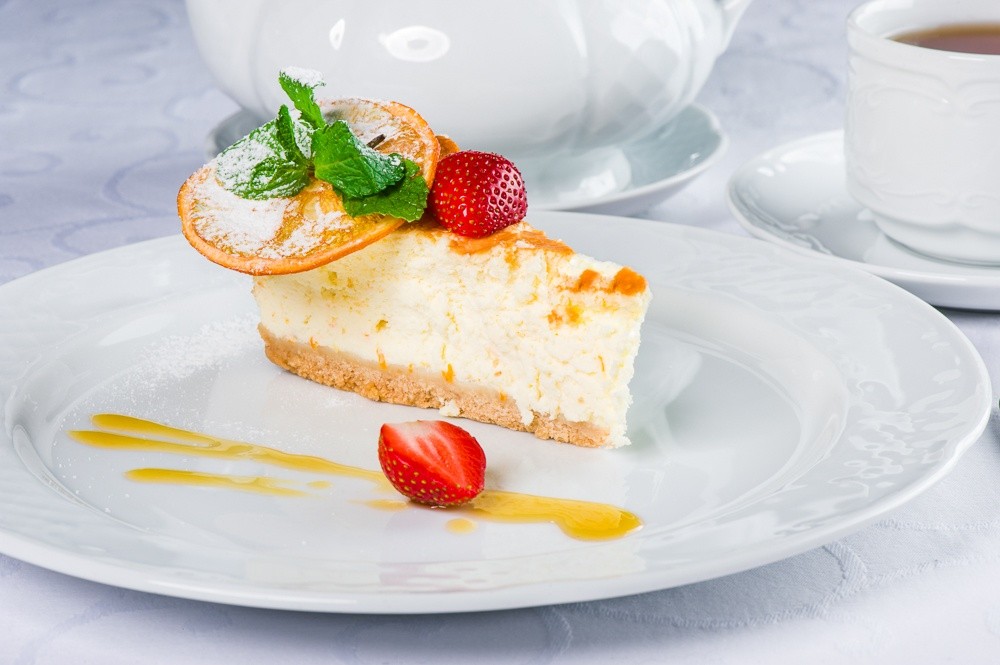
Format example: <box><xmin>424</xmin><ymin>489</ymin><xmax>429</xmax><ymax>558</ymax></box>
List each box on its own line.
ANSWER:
<box><xmin>0</xmin><ymin>204</ymin><xmax>996</xmax><ymax>618</ymax></box>
<box><xmin>204</xmin><ymin>97</ymin><xmax>727</xmax><ymax>225</ymax></box>
<box><xmin>723</xmin><ymin>131</ymin><xmax>1000</xmax><ymax>324</ymax></box>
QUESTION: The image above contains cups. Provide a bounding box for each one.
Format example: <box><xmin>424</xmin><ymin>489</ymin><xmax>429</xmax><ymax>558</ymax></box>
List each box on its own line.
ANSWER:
<box><xmin>844</xmin><ymin>2</ymin><xmax>1000</xmax><ymax>258</ymax></box>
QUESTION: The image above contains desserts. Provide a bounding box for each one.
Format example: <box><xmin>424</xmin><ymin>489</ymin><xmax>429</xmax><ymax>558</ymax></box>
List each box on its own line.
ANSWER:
<box><xmin>175</xmin><ymin>67</ymin><xmax>655</xmax><ymax>506</ymax></box>
